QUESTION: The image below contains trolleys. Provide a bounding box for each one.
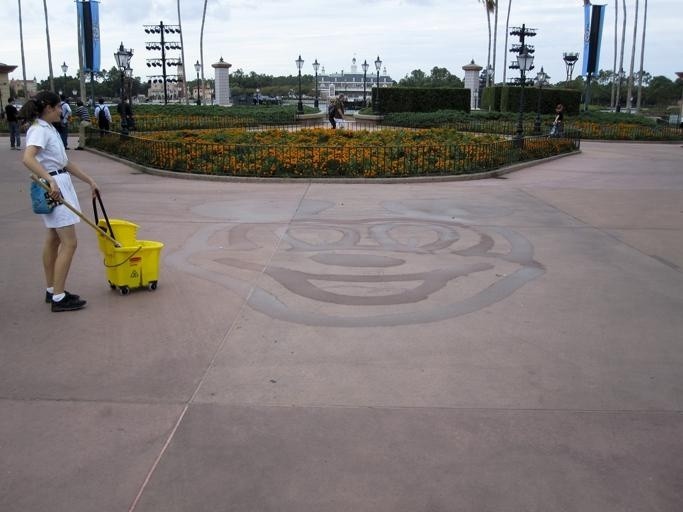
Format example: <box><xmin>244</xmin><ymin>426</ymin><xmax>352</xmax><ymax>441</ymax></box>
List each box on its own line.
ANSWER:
<box><xmin>91</xmin><ymin>188</ymin><xmax>165</xmax><ymax>295</ymax></box>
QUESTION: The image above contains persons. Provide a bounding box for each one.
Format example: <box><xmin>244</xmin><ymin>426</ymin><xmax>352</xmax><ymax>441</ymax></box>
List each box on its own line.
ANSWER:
<box><xmin>328</xmin><ymin>94</ymin><xmax>345</xmax><ymax>129</ymax></box>
<box><xmin>547</xmin><ymin>104</ymin><xmax>563</xmax><ymax>141</ymax></box>
<box><xmin>94</xmin><ymin>98</ymin><xmax>113</xmax><ymax>137</ymax></box>
<box><xmin>5</xmin><ymin>95</ymin><xmax>91</xmax><ymax>150</ymax></box>
<box><xmin>117</xmin><ymin>95</ymin><xmax>134</xmax><ymax>130</ymax></box>
<box><xmin>17</xmin><ymin>91</ymin><xmax>101</xmax><ymax>312</ymax></box>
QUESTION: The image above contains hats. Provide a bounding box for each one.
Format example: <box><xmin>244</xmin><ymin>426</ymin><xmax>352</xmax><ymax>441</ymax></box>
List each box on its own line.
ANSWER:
<box><xmin>555</xmin><ymin>104</ymin><xmax>564</xmax><ymax>111</ymax></box>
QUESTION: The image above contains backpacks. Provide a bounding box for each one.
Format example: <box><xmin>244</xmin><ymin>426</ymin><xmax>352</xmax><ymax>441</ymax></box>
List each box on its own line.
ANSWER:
<box><xmin>53</xmin><ymin>103</ymin><xmax>68</xmax><ymax>134</ymax></box>
<box><xmin>98</xmin><ymin>106</ymin><xmax>107</xmax><ymax>121</ymax></box>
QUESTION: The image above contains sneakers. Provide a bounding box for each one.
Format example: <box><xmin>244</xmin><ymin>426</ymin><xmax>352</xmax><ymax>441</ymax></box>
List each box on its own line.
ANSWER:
<box><xmin>45</xmin><ymin>291</ymin><xmax>88</xmax><ymax>312</ymax></box>
<box><xmin>10</xmin><ymin>146</ymin><xmax>23</xmax><ymax>150</ymax></box>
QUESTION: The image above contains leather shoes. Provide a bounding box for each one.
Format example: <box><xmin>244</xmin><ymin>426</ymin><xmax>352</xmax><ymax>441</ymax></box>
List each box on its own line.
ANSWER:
<box><xmin>74</xmin><ymin>147</ymin><xmax>84</xmax><ymax>150</ymax></box>
<box><xmin>65</xmin><ymin>147</ymin><xmax>70</xmax><ymax>150</ymax></box>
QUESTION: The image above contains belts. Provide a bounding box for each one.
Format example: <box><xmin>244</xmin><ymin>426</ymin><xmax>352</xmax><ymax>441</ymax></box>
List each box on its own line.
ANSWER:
<box><xmin>81</xmin><ymin>119</ymin><xmax>89</xmax><ymax>121</ymax></box>
<box><xmin>38</xmin><ymin>168</ymin><xmax>67</xmax><ymax>179</ymax></box>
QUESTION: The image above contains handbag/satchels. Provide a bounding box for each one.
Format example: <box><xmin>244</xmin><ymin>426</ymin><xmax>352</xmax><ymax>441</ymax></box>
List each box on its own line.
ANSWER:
<box><xmin>31</xmin><ymin>178</ymin><xmax>54</xmax><ymax>214</ymax></box>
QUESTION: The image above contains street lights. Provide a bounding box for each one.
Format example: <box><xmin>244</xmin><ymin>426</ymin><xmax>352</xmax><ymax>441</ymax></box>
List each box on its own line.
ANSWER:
<box><xmin>71</xmin><ymin>88</ymin><xmax>77</xmax><ymax>105</ymax></box>
<box><xmin>510</xmin><ymin>44</ymin><xmax>534</xmax><ymax>151</ymax></box>
<box><xmin>61</xmin><ymin>61</ymin><xmax>68</xmax><ymax>96</ymax></box>
<box><xmin>255</xmin><ymin>87</ymin><xmax>260</xmax><ymax>104</ymax></box>
<box><xmin>57</xmin><ymin>88</ymin><xmax>62</xmax><ymax>95</ymax></box>
<box><xmin>192</xmin><ymin>58</ymin><xmax>202</xmax><ymax>106</ymax></box>
<box><xmin>373</xmin><ymin>56</ymin><xmax>381</xmax><ymax>115</ymax></box>
<box><xmin>360</xmin><ymin>59</ymin><xmax>369</xmax><ymax>106</ymax></box>
<box><xmin>124</xmin><ymin>63</ymin><xmax>132</xmax><ymax>105</ymax></box>
<box><xmin>531</xmin><ymin>66</ymin><xmax>547</xmax><ymax>136</ymax></box>
<box><xmin>294</xmin><ymin>55</ymin><xmax>304</xmax><ymax>115</ymax></box>
<box><xmin>113</xmin><ymin>40</ymin><xmax>130</xmax><ymax>142</ymax></box>
<box><xmin>311</xmin><ymin>57</ymin><xmax>320</xmax><ymax>107</ymax></box>
